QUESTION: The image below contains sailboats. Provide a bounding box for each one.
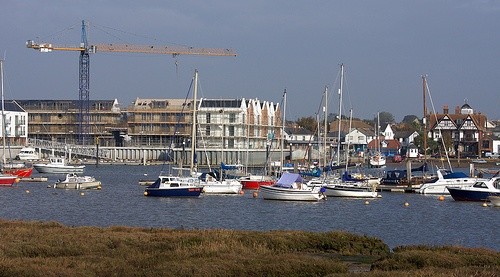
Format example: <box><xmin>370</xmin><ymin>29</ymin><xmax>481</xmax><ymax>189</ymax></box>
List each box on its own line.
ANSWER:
<box><xmin>139</xmin><ymin>67</ymin><xmax>244</xmax><ymax>197</ymax></box>
<box><xmin>268</xmin><ymin>88</ymin><xmax>296</xmax><ymax>178</ymax></box>
<box><xmin>307</xmin><ymin>63</ymin><xmax>500</xmax><ymax>206</ymax></box>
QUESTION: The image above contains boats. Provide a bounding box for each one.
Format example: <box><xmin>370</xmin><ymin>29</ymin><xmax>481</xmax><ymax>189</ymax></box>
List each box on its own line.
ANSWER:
<box><xmin>238</xmin><ymin>175</ymin><xmax>277</xmax><ymax>190</ymax></box>
<box><xmin>219</xmin><ymin>163</ymin><xmax>252</xmax><ymax>177</ymax></box>
<box><xmin>56</xmin><ymin>174</ymin><xmax>101</xmax><ymax>189</ymax></box>
<box><xmin>259</xmin><ymin>171</ymin><xmax>328</xmax><ymax>202</ymax></box>
<box><xmin>0</xmin><ymin>145</ymin><xmax>43</xmax><ymax>186</ymax></box>
<box><xmin>33</xmin><ymin>158</ymin><xmax>86</xmax><ymax>174</ymax></box>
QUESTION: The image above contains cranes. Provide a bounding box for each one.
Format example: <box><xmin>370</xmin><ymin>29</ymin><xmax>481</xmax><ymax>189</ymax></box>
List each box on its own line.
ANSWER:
<box><xmin>24</xmin><ymin>20</ymin><xmax>241</xmax><ymax>143</ymax></box>
